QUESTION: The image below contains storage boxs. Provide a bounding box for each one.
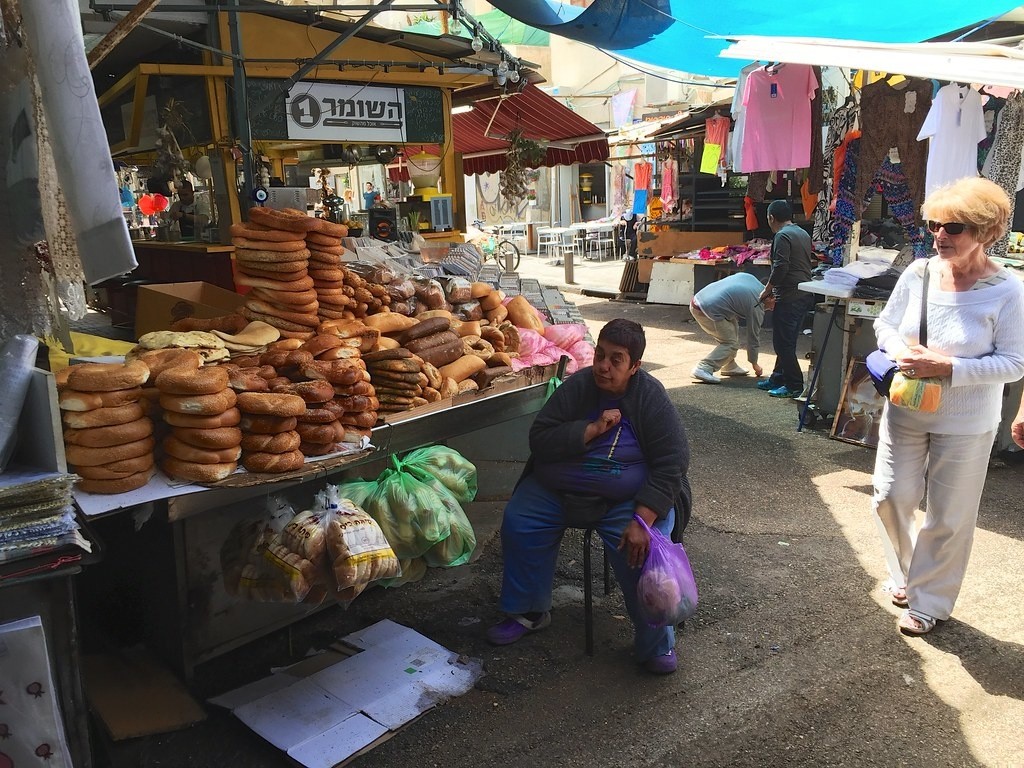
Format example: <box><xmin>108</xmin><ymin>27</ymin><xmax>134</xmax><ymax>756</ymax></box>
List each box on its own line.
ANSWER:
<box><xmin>133</xmin><ymin>281</ymin><xmax>245</xmax><ymax>340</ymax></box>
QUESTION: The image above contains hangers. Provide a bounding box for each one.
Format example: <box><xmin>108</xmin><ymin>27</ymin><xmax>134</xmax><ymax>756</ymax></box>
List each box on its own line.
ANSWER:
<box><xmin>977</xmin><ymin>85</ymin><xmax>997</xmax><ymax>99</ymax></box>
<box><xmin>662</xmin><ymin>154</ymin><xmax>677</xmax><ymax>164</ymax></box>
<box><xmin>613</xmin><ymin>159</ymin><xmax>623</xmax><ymax>168</ymax></box>
<box><xmin>704</xmin><ymin>110</ymin><xmax>728</xmax><ymax>120</ymax></box>
<box><xmin>637</xmin><ymin>156</ymin><xmax>651</xmax><ymax>165</ymax></box>
<box><xmin>883</xmin><ymin>73</ymin><xmax>912</xmax><ymax>83</ymax></box>
<box><xmin>833</xmin><ymin>85</ymin><xmax>859</xmax><ymax>116</ymax></box>
<box><xmin>956</xmin><ymin>82</ymin><xmax>970</xmax><ymax>92</ymax></box>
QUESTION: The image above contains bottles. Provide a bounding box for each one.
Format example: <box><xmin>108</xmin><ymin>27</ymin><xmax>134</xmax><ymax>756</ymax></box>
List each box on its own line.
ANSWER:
<box><xmin>646</xmin><ymin>189</ymin><xmax>668</xmax><ymax>232</ymax></box>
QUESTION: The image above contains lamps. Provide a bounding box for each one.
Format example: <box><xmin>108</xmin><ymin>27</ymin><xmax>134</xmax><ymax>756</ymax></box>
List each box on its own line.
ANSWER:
<box><xmin>509</xmin><ymin>64</ymin><xmax>519</xmax><ymax>83</ymax></box>
<box><xmin>471</xmin><ymin>28</ymin><xmax>483</xmax><ymax>51</ymax></box>
<box><xmin>497</xmin><ymin>71</ymin><xmax>505</xmax><ymax>85</ymax></box>
<box><xmin>498</xmin><ymin>53</ymin><xmax>508</xmax><ymax>74</ymax></box>
<box><xmin>450</xmin><ymin>10</ymin><xmax>462</xmax><ymax>36</ymax></box>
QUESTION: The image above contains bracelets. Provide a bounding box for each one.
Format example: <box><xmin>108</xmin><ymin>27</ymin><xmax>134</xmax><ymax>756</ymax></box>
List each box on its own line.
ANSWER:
<box><xmin>182</xmin><ymin>211</ymin><xmax>186</xmax><ymax>218</ymax></box>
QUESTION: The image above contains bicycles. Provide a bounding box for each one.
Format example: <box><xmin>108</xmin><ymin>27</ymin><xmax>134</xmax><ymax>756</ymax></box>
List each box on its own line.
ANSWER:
<box><xmin>472</xmin><ymin>219</ymin><xmax>521</xmax><ymax>270</ymax></box>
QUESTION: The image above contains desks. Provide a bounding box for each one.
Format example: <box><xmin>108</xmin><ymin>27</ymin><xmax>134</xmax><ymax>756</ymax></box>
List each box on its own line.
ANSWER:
<box><xmin>77</xmin><ymin>377</ymin><xmax>567</xmax><ymax>691</ymax></box>
<box><xmin>105</xmin><ymin>240</ymin><xmax>251</xmax><ymax>327</ymax></box>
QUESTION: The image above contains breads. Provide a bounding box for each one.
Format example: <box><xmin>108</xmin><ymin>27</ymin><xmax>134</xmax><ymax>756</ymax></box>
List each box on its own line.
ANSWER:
<box><xmin>213</xmin><ymin>452</ymin><xmax>476</xmax><ymax>605</ymax></box>
<box><xmin>51</xmin><ymin>315</ymin><xmax>379</xmax><ymax>494</ymax></box>
<box><xmin>228</xmin><ymin>206</ymin><xmax>594</xmax><ymax>412</ymax></box>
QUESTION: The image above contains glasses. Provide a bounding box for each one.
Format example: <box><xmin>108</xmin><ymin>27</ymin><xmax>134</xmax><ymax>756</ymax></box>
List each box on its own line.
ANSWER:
<box><xmin>928</xmin><ymin>220</ymin><xmax>976</xmax><ymax>235</ymax></box>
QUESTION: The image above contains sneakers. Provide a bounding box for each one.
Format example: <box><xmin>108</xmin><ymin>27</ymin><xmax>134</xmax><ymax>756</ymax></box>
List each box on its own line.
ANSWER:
<box><xmin>757</xmin><ymin>379</ymin><xmax>785</xmax><ymax>390</ymax></box>
<box><xmin>690</xmin><ymin>365</ymin><xmax>721</xmax><ymax>383</ymax></box>
<box><xmin>768</xmin><ymin>386</ymin><xmax>803</xmax><ymax>397</ymax></box>
<box><xmin>721</xmin><ymin>366</ymin><xmax>749</xmax><ymax>375</ymax></box>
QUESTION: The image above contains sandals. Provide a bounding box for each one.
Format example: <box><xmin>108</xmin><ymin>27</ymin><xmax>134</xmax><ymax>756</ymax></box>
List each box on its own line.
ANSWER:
<box><xmin>646</xmin><ymin>647</ymin><xmax>678</xmax><ymax>674</ymax></box>
<box><xmin>487</xmin><ymin>611</ymin><xmax>551</xmax><ymax>645</ymax></box>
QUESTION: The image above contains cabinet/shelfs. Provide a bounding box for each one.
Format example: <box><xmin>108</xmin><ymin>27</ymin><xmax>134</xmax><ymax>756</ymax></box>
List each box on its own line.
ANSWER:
<box><xmin>418</xmin><ymin>229</ymin><xmax>461</xmax><ymax>243</ymax></box>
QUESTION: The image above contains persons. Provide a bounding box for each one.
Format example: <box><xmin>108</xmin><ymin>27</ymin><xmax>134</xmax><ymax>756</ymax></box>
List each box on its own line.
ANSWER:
<box><xmin>757</xmin><ymin>200</ymin><xmax>813</xmax><ymax>397</ymax></box>
<box><xmin>619</xmin><ymin>208</ymin><xmax>642</xmax><ymax>261</ymax></box>
<box><xmin>159</xmin><ymin>180</ymin><xmax>210</xmax><ymax>239</ymax></box>
<box><xmin>689</xmin><ymin>272</ymin><xmax>776</xmax><ymax>383</ymax></box>
<box><xmin>363</xmin><ymin>182</ymin><xmax>379</xmax><ymax>210</ymax></box>
<box><xmin>872</xmin><ymin>176</ymin><xmax>1024</xmax><ymax>633</ymax></box>
<box><xmin>486</xmin><ymin>319</ymin><xmax>693</xmax><ymax>673</ymax></box>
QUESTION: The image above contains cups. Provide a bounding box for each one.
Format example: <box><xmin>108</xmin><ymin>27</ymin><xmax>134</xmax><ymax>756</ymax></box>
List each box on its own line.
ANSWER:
<box><xmin>157</xmin><ymin>226</ymin><xmax>170</xmax><ymax>242</ymax></box>
<box><xmin>129</xmin><ymin>229</ymin><xmax>139</xmax><ymax>239</ymax></box>
<box><xmin>343</xmin><ymin>204</ymin><xmax>351</xmax><ymax>222</ymax></box>
<box><xmin>168</xmin><ymin>230</ymin><xmax>180</xmax><ymax>241</ymax></box>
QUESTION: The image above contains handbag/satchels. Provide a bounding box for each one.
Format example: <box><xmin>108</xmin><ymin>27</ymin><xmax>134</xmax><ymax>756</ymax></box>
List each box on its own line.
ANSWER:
<box><xmin>866</xmin><ymin>348</ymin><xmax>900</xmax><ymax>398</ymax></box>
<box><xmin>633</xmin><ymin>513</ymin><xmax>701</xmax><ymax>631</ymax></box>
<box><xmin>888</xmin><ymin>371</ymin><xmax>944</xmax><ymax>413</ymax></box>
<box><xmin>219</xmin><ymin>444</ymin><xmax>478</xmax><ymax>613</ymax></box>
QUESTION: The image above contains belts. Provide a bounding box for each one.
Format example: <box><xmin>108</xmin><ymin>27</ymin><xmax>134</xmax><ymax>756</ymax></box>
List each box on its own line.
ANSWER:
<box><xmin>692</xmin><ymin>300</ymin><xmax>701</xmax><ymax>311</ymax></box>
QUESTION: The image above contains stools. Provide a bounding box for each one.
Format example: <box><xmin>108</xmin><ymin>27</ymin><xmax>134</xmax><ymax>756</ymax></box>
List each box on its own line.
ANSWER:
<box><xmin>583</xmin><ymin>527</ymin><xmax>684</xmax><ymax>658</ymax></box>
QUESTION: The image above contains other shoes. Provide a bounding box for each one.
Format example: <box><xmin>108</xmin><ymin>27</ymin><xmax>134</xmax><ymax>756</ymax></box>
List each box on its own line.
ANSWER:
<box><xmin>892</xmin><ymin>584</ymin><xmax>909</xmax><ymax>605</ymax></box>
<box><xmin>899</xmin><ymin>609</ymin><xmax>938</xmax><ymax>634</ymax></box>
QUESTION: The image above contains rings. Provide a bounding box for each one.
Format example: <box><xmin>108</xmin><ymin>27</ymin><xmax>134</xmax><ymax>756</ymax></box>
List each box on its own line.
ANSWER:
<box><xmin>906</xmin><ymin>370</ymin><xmax>915</xmax><ymax>376</ymax></box>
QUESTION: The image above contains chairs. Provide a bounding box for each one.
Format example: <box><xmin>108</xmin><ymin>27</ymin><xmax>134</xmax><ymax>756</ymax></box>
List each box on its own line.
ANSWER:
<box><xmin>494</xmin><ymin>217</ymin><xmax>626</xmax><ymax>262</ymax></box>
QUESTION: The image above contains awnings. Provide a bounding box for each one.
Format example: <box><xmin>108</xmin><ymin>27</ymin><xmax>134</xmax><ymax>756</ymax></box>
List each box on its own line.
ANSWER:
<box><xmin>384</xmin><ymin>83</ymin><xmax>610</xmax><ymax>183</ymax></box>
